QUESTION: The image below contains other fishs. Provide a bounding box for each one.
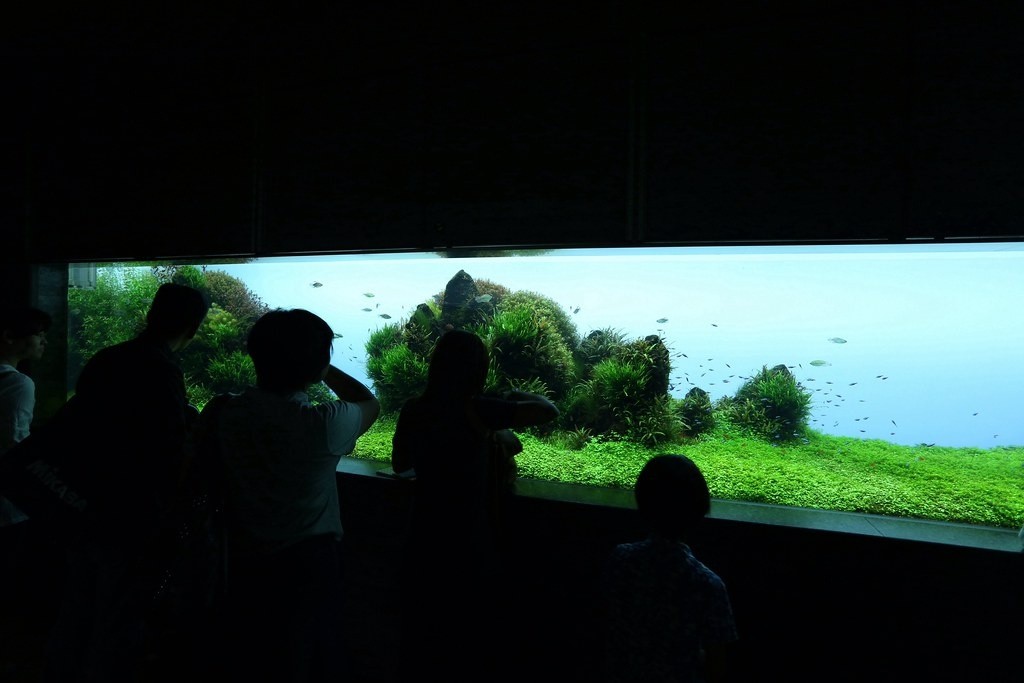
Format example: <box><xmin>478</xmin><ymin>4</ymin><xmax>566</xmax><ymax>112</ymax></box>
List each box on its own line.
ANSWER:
<box><xmin>311</xmin><ymin>276</ymin><xmax>901</xmax><ymax>441</ymax></box>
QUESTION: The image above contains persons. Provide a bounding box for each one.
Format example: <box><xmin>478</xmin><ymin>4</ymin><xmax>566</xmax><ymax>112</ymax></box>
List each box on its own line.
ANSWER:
<box><xmin>584</xmin><ymin>455</ymin><xmax>740</xmax><ymax>683</ymax></box>
<box><xmin>43</xmin><ymin>283</ymin><xmax>206</xmax><ymax>683</ymax></box>
<box><xmin>393</xmin><ymin>331</ymin><xmax>558</xmax><ymax>683</ymax></box>
<box><xmin>0</xmin><ymin>303</ymin><xmax>52</xmax><ymax>455</ymax></box>
<box><xmin>189</xmin><ymin>310</ymin><xmax>380</xmax><ymax>683</ymax></box>
